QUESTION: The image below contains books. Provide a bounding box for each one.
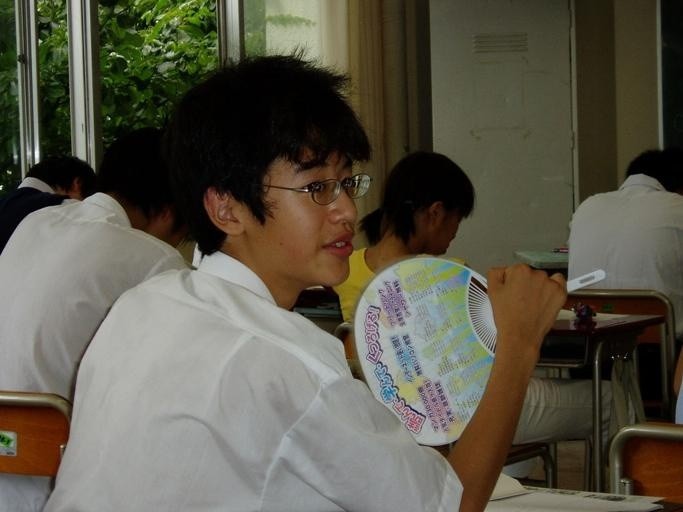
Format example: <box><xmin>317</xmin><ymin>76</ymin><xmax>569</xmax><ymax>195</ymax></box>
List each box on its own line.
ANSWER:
<box><xmin>482</xmin><ymin>486</ymin><xmax>666</xmax><ymax>512</ymax></box>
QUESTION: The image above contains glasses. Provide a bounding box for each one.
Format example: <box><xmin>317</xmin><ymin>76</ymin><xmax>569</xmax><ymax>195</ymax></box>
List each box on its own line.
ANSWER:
<box><xmin>255</xmin><ymin>171</ymin><xmax>374</xmax><ymax>207</ymax></box>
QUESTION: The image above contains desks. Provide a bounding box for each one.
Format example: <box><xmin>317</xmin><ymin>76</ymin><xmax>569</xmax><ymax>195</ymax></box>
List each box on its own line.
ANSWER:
<box><xmin>514</xmin><ymin>247</ymin><xmax>569</xmax><ymax>270</ymax></box>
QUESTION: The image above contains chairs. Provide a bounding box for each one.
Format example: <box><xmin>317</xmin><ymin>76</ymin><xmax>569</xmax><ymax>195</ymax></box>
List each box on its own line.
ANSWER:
<box><xmin>0</xmin><ymin>278</ymin><xmax>683</xmax><ymax>512</ymax></box>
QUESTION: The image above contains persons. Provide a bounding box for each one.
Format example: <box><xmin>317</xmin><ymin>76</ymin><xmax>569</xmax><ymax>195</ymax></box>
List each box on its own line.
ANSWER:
<box><xmin>0</xmin><ymin>152</ymin><xmax>95</xmax><ymax>259</ymax></box>
<box><xmin>0</xmin><ymin>125</ymin><xmax>189</xmax><ymax>512</ymax></box>
<box><xmin>41</xmin><ymin>48</ymin><xmax>569</xmax><ymax>512</ymax></box>
<box><xmin>567</xmin><ymin>148</ymin><xmax>683</xmax><ymax>402</ymax></box>
<box><xmin>340</xmin><ymin>151</ymin><xmax>631</xmax><ymax>452</ymax></box>
